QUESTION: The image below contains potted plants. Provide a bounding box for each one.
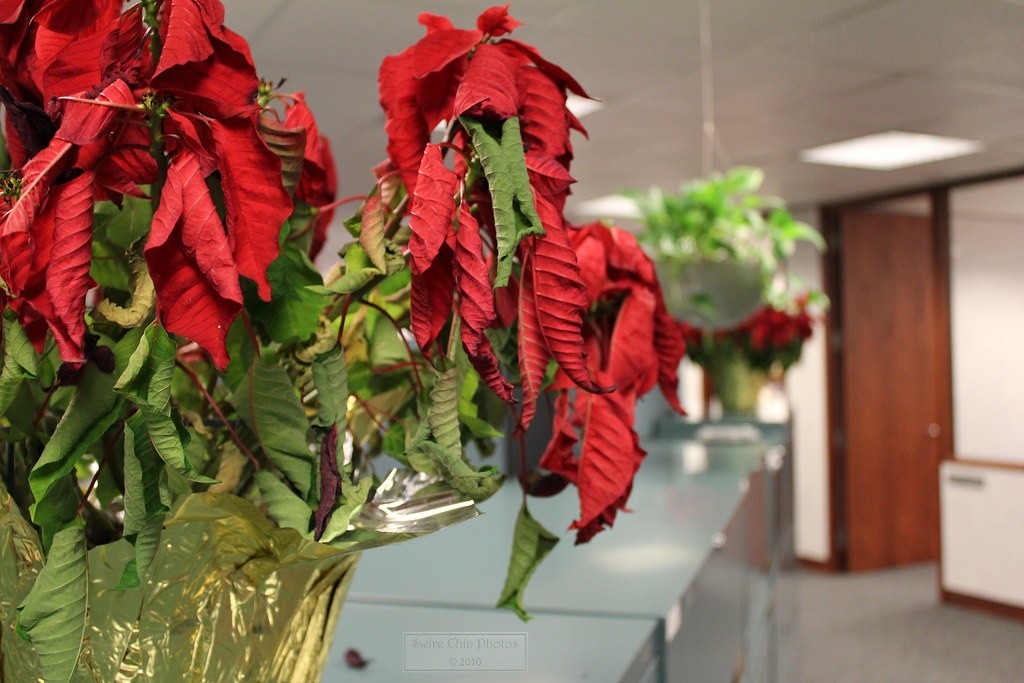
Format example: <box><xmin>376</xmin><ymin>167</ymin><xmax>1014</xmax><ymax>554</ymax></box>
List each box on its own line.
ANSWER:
<box><xmin>0</xmin><ymin>0</ymin><xmax>687</xmax><ymax>683</ymax></box>
<box><xmin>630</xmin><ymin>167</ymin><xmax>830</xmax><ymax>444</ymax></box>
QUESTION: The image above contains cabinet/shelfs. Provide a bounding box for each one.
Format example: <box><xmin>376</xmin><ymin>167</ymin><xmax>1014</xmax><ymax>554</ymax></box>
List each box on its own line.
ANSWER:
<box><xmin>316</xmin><ymin>421</ymin><xmax>791</xmax><ymax>683</ymax></box>
